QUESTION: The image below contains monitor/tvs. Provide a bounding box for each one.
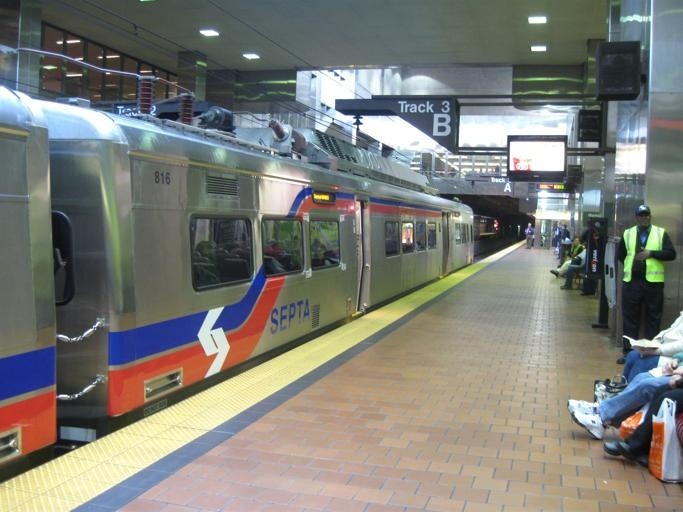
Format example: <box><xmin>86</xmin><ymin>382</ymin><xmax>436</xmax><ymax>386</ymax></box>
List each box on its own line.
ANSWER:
<box><xmin>507</xmin><ymin>135</ymin><xmax>567</xmax><ymax>182</ymax></box>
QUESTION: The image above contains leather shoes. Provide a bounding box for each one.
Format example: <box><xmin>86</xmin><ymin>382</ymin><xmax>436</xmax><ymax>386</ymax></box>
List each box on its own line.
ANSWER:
<box><xmin>616</xmin><ymin>442</ymin><xmax>636</xmax><ymax>461</ymax></box>
<box><xmin>603</xmin><ymin>441</ymin><xmax>622</xmax><ymax>456</ymax></box>
<box><xmin>616</xmin><ymin>356</ymin><xmax>627</xmax><ymax>364</ymax></box>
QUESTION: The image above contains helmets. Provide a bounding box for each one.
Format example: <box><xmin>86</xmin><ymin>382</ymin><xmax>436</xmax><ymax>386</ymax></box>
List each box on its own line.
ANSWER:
<box><xmin>635</xmin><ymin>205</ymin><xmax>650</xmax><ymax>216</ymax></box>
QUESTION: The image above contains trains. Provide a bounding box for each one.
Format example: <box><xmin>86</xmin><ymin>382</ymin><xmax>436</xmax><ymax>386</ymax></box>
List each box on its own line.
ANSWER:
<box><xmin>0</xmin><ymin>45</ymin><xmax>474</xmax><ymax>483</ymax></box>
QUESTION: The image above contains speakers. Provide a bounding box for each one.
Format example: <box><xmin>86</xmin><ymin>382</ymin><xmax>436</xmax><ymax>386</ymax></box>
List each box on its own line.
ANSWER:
<box><xmin>596</xmin><ymin>41</ymin><xmax>640</xmax><ymax>100</ymax></box>
<box><xmin>578</xmin><ymin>109</ymin><xmax>601</xmax><ymax>141</ymax></box>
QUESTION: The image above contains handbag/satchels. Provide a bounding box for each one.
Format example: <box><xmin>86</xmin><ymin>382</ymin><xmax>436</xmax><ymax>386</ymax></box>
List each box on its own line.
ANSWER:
<box><xmin>594</xmin><ymin>375</ymin><xmax>628</xmax><ymax>404</ymax></box>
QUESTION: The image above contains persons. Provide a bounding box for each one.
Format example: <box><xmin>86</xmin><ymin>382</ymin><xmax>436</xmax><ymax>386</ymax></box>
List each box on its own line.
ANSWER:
<box><xmin>616</xmin><ymin>204</ymin><xmax>675</xmax><ymax>364</ymax></box>
<box><xmin>184</xmin><ymin>221</ymin><xmax>341</xmax><ymax>286</ymax></box>
<box><xmin>566</xmin><ymin>310</ymin><xmax>683</xmax><ymax>463</ymax></box>
<box><xmin>524</xmin><ymin>221</ymin><xmax>600</xmax><ymax>296</ymax></box>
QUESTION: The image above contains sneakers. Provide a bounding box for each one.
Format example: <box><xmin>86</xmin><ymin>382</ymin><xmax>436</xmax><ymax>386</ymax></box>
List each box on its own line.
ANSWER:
<box><xmin>567</xmin><ymin>399</ymin><xmax>600</xmax><ymax>416</ymax></box>
<box><xmin>550</xmin><ymin>269</ymin><xmax>559</xmax><ymax>276</ymax></box>
<box><xmin>560</xmin><ymin>285</ymin><xmax>572</xmax><ymax>289</ymax></box>
<box><xmin>571</xmin><ymin>411</ymin><xmax>606</xmax><ymax>440</ymax></box>
